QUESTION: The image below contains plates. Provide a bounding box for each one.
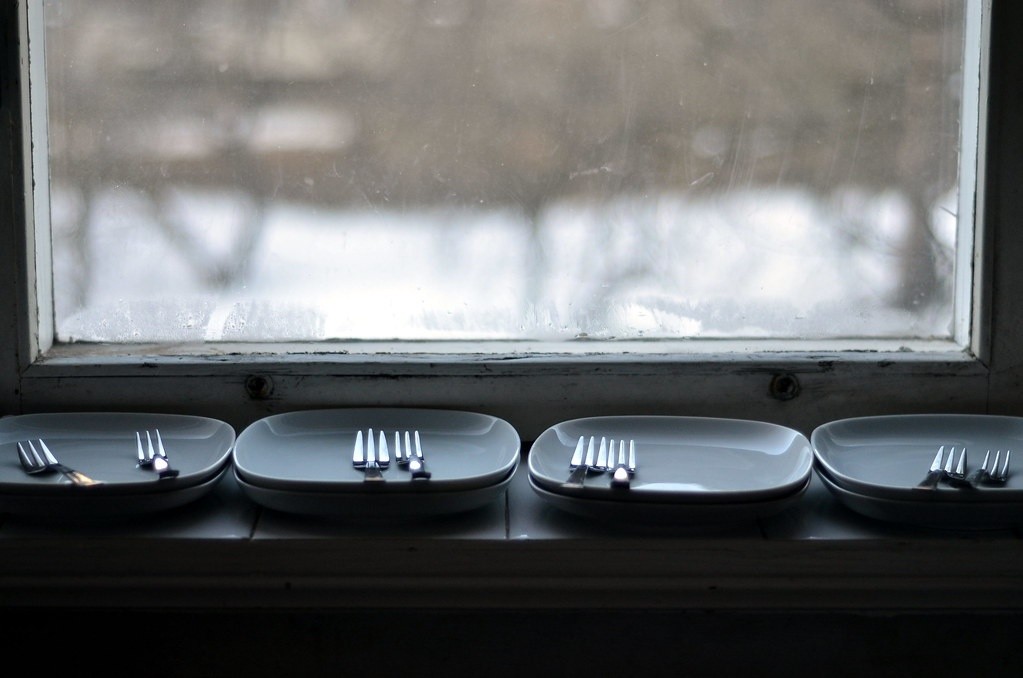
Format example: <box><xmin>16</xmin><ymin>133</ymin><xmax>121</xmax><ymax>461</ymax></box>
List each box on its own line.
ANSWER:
<box><xmin>0</xmin><ymin>408</ymin><xmax>1023</xmax><ymax>529</ymax></box>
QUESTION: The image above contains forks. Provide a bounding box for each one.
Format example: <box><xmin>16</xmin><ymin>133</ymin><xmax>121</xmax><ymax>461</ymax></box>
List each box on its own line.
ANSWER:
<box><xmin>395</xmin><ymin>430</ymin><xmax>432</xmax><ymax>480</ymax></box>
<box><xmin>353</xmin><ymin>426</ymin><xmax>391</xmax><ymax>483</ymax></box>
<box><xmin>608</xmin><ymin>439</ymin><xmax>637</xmax><ymax>489</ymax></box>
<box><xmin>135</xmin><ymin>427</ymin><xmax>180</xmax><ymax>478</ymax></box>
<box><xmin>913</xmin><ymin>445</ymin><xmax>967</xmax><ymax>492</ymax></box>
<box><xmin>964</xmin><ymin>449</ymin><xmax>1012</xmax><ymax>491</ymax></box>
<box><xmin>560</xmin><ymin>435</ymin><xmax>606</xmax><ymax>489</ymax></box>
<box><xmin>15</xmin><ymin>438</ymin><xmax>106</xmax><ymax>487</ymax></box>
